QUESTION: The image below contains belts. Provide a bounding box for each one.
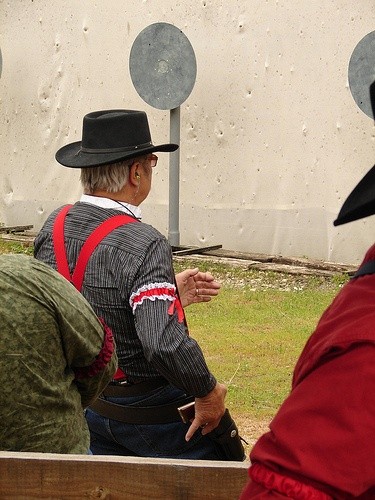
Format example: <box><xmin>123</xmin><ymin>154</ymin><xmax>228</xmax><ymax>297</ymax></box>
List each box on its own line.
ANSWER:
<box><xmin>89</xmin><ymin>396</ymin><xmax>195</xmax><ymax>423</ymax></box>
<box><xmin>99</xmin><ymin>379</ymin><xmax>167</xmax><ymax>398</ymax></box>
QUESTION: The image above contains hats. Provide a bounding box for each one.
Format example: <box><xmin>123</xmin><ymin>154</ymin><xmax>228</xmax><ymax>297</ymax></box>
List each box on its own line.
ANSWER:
<box><xmin>55</xmin><ymin>109</ymin><xmax>179</xmax><ymax>168</ymax></box>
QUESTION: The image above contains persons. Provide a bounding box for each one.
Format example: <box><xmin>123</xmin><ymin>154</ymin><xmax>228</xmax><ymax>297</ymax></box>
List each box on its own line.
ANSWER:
<box><xmin>33</xmin><ymin>111</ymin><xmax>247</xmax><ymax>462</ymax></box>
<box><xmin>236</xmin><ymin>79</ymin><xmax>375</xmax><ymax>500</ymax></box>
<box><xmin>0</xmin><ymin>252</ymin><xmax>118</xmax><ymax>455</ymax></box>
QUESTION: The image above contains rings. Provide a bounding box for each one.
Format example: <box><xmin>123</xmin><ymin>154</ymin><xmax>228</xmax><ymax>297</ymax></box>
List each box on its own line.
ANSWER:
<box><xmin>195</xmin><ymin>288</ymin><xmax>199</xmax><ymax>296</ymax></box>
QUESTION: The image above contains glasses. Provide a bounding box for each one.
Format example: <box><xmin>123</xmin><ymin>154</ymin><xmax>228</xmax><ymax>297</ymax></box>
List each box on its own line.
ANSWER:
<box><xmin>135</xmin><ymin>154</ymin><xmax>158</xmax><ymax>167</ymax></box>
